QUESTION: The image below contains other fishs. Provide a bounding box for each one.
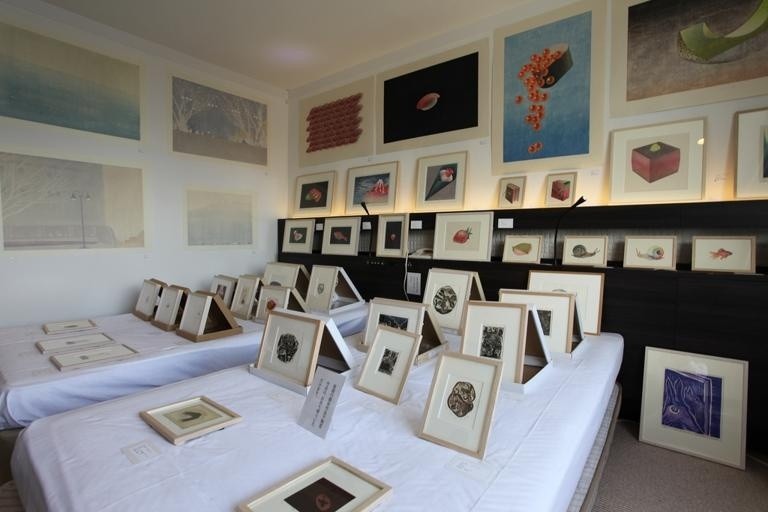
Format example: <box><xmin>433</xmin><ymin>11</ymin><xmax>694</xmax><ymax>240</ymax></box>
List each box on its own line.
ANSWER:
<box><xmin>710</xmin><ymin>248</ymin><xmax>733</xmax><ymax>260</ymax></box>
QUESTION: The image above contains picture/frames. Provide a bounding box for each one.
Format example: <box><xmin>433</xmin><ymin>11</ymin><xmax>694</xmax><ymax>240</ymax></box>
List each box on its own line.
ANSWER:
<box><xmin>561</xmin><ymin>235</ymin><xmax>609</xmax><ymax>269</ymax></box>
<box><xmin>498</xmin><ymin>288</ymin><xmax>586</xmax><ymax>361</ymax></box>
<box><xmin>282</xmin><ymin>218</ymin><xmax>314</xmax><ymax>253</ymax></box>
<box><xmin>431</xmin><ymin>212</ymin><xmax>494</xmax><ymax>262</ymax></box>
<box><xmin>36</xmin><ymin>319</ymin><xmax>140</xmax><ymax>372</ymax></box>
<box><xmin>230</xmin><ymin>263</ymin><xmax>365</xmax><ymax>324</ymax></box>
<box><xmin>375</xmin><ymin>212</ymin><xmax>410</xmax><ymax>258</ymax></box>
<box><xmin>733</xmin><ymin>107</ymin><xmax>768</xmax><ymax>200</ymax></box>
<box><xmin>134</xmin><ymin>274</ymin><xmax>243</xmax><ymax>341</ymax></box>
<box><xmin>297</xmin><ymin>75</ymin><xmax>374</xmax><ymax>167</ymax></box>
<box><xmin>376</xmin><ymin>37</ymin><xmax>490</xmax><ymax>155</ymax></box>
<box><xmin>138</xmin><ymin>395</ymin><xmax>243</xmax><ymax>446</ymax></box>
<box><xmin>357</xmin><ymin>297</ymin><xmax>449</xmax><ymax>367</ymax></box>
<box><xmin>498</xmin><ymin>176</ymin><xmax>528</xmax><ymax>210</ymax></box>
<box><xmin>608</xmin><ymin>116</ymin><xmax>708</xmax><ymax>206</ymax></box>
<box><xmin>236</xmin><ymin>456</ymin><xmax>393</xmax><ymax>511</ymax></box>
<box><xmin>414</xmin><ymin>150</ymin><xmax>468</xmax><ymax>213</ymax></box>
<box><xmin>503</xmin><ymin>234</ymin><xmax>542</xmax><ymax>264</ymax></box>
<box><xmin>321</xmin><ymin>217</ymin><xmax>361</xmax><ymax>255</ymax></box>
<box><xmin>609</xmin><ymin>1</ymin><xmax>766</xmax><ymax>119</ymax></box>
<box><xmin>527</xmin><ymin>270</ymin><xmax>604</xmax><ymax>336</ymax></box>
<box><xmin>423</xmin><ymin>267</ymin><xmax>485</xmax><ymax>337</ymax></box>
<box><xmin>354</xmin><ymin>323</ymin><xmax>423</xmax><ymax>406</ymax></box>
<box><xmin>250</xmin><ymin>308</ymin><xmax>360</xmax><ymax>399</ymax></box>
<box><xmin>691</xmin><ymin>235</ymin><xmax>755</xmax><ymax>272</ymax></box>
<box><xmin>293</xmin><ymin>170</ymin><xmax>336</xmax><ymax>217</ymax></box>
<box><xmin>634</xmin><ymin>339</ymin><xmax>750</xmax><ymax>474</ymax></box>
<box><xmin>622</xmin><ymin>235</ymin><xmax>677</xmax><ymax>271</ymax></box>
<box><xmin>417</xmin><ymin>349</ymin><xmax>504</xmax><ymax>461</ymax></box>
<box><xmin>492</xmin><ymin>0</ymin><xmax>605</xmax><ymax>177</ymax></box>
<box><xmin>460</xmin><ymin>299</ymin><xmax>553</xmax><ymax>394</ymax></box>
<box><xmin>345</xmin><ymin>161</ymin><xmax>400</xmax><ymax>216</ymax></box>
<box><xmin>545</xmin><ymin>172</ymin><xmax>576</xmax><ymax>208</ymax></box>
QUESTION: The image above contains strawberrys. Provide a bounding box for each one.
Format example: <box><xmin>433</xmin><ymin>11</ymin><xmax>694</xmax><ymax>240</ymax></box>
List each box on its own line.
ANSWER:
<box><xmin>453</xmin><ymin>226</ymin><xmax>472</xmax><ymax>243</ymax></box>
